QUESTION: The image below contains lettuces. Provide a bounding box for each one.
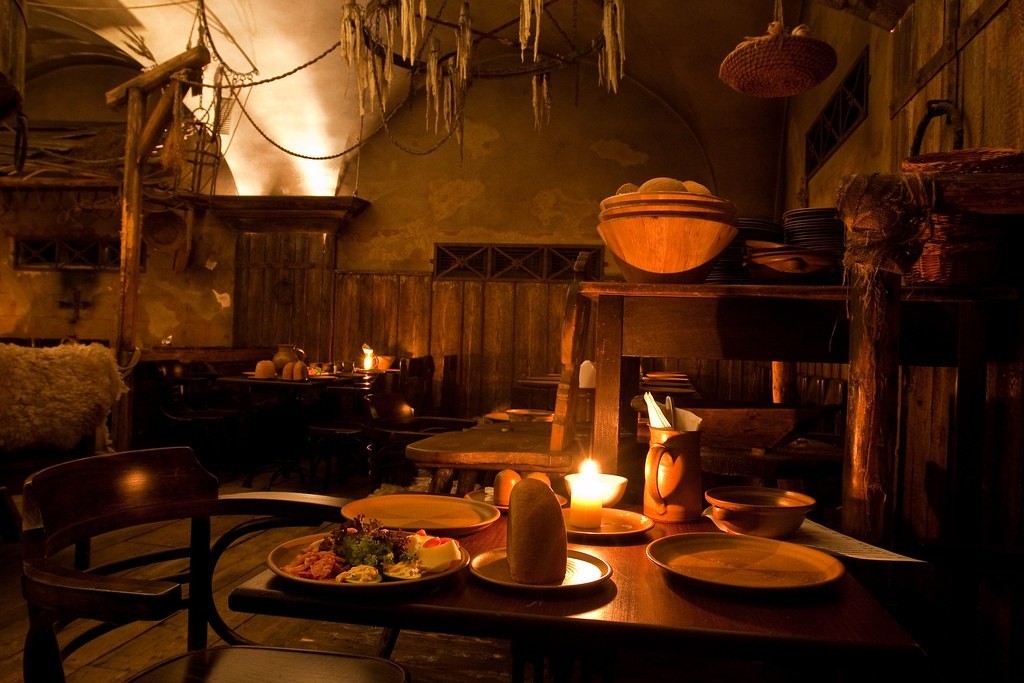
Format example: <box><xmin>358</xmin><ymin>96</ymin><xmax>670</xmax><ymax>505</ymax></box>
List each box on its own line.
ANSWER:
<box><xmin>319</xmin><ymin>513</ymin><xmax>412</xmax><ymax>566</ymax></box>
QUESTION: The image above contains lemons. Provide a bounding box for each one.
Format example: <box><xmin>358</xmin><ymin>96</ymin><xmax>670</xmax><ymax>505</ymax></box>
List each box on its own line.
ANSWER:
<box><xmin>255</xmin><ymin>361</ymin><xmax>274</xmax><ymax>378</ymax></box>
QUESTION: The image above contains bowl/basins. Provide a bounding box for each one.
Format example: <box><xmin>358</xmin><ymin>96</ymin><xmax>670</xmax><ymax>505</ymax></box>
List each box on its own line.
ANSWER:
<box><xmin>372</xmin><ymin>356</ymin><xmax>397</xmax><ymax>370</ymax></box>
<box><xmin>566</xmin><ymin>473</ymin><xmax>628</xmax><ymax>506</ymax></box>
<box><xmin>702</xmin><ymin>487</ymin><xmax>817</xmax><ymax>538</ymax></box>
<box><xmin>596</xmin><ymin>193</ymin><xmax>737</xmax><ymax>285</ymax></box>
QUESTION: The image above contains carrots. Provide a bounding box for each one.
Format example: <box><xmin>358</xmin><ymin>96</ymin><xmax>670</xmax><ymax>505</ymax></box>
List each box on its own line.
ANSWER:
<box><xmin>416</xmin><ymin>529</ymin><xmax>460</xmax><ymax>547</ymax></box>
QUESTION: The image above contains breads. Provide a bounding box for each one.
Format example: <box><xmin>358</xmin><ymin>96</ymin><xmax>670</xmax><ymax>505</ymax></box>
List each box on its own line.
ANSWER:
<box><xmin>283</xmin><ymin>362</ymin><xmax>308</xmax><ymax>379</ymax></box>
<box><xmin>494</xmin><ymin>468</ymin><xmax>567</xmax><ymax>585</ymax></box>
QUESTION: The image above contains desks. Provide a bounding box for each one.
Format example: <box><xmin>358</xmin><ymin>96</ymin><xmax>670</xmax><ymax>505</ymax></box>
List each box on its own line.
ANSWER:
<box><xmin>210</xmin><ymin>369</ymin><xmax>400</xmax><ymax>488</ymax></box>
<box><xmin>397</xmin><ymin>417</ymin><xmax>634</xmax><ymax>499</ymax></box>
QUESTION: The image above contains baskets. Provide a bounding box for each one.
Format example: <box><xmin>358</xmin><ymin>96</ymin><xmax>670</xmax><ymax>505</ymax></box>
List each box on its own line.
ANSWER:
<box><xmin>719</xmin><ymin>33</ymin><xmax>837</xmax><ymax>98</ymax></box>
<box><xmin>900</xmin><ymin>146</ymin><xmax>1024</xmax><ymax>285</ymax></box>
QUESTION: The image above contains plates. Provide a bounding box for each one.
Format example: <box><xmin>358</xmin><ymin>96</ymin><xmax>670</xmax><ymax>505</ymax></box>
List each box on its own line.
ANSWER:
<box><xmin>468</xmin><ymin>545</ymin><xmax>613</xmax><ymax>591</ymax></box>
<box><xmin>279</xmin><ymin>378</ymin><xmax>310</xmax><ymax>383</ymax></box>
<box><xmin>268</xmin><ymin>527</ymin><xmax>470</xmax><ymax>590</ymax></box>
<box><xmin>705</xmin><ymin>205</ymin><xmax>841</xmax><ymax>283</ymax></box>
<box><xmin>309</xmin><ymin>374</ymin><xmax>336</xmax><ymax>380</ymax></box>
<box><xmin>248</xmin><ymin>376</ymin><xmax>280</xmax><ymax>380</ymax></box>
<box><xmin>341</xmin><ymin>494</ymin><xmax>501</xmax><ymax>537</ymax></box>
<box><xmin>555</xmin><ymin>505</ymin><xmax>655</xmax><ymax>536</ymax></box>
<box><xmin>464</xmin><ymin>487</ymin><xmax>567</xmax><ymax>511</ymax></box>
<box><xmin>645</xmin><ymin>531</ymin><xmax>845</xmax><ymax>598</ymax></box>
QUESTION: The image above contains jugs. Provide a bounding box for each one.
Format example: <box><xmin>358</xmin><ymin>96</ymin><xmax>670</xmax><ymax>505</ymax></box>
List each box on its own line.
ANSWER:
<box><xmin>272</xmin><ymin>344</ymin><xmax>307</xmax><ymax>372</ymax></box>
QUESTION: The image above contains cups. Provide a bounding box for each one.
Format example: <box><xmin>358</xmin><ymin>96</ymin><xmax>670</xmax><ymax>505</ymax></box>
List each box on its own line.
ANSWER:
<box><xmin>643</xmin><ymin>423</ymin><xmax>703</xmax><ymax>523</ymax></box>
<box><xmin>310</xmin><ymin>361</ymin><xmax>345</xmax><ymax>374</ymax></box>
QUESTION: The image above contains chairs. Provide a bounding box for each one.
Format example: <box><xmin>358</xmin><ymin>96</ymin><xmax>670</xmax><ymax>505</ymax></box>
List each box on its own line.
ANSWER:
<box><xmin>139</xmin><ymin>350</ymin><xmax>479</xmax><ymax>496</ymax></box>
<box><xmin>18</xmin><ymin>444</ymin><xmax>408</xmax><ymax>683</ymax></box>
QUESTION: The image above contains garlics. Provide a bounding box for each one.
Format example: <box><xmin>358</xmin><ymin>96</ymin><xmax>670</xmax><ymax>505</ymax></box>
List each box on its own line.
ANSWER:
<box><xmin>767</xmin><ymin>21</ymin><xmax>810</xmax><ymax>36</ymax></box>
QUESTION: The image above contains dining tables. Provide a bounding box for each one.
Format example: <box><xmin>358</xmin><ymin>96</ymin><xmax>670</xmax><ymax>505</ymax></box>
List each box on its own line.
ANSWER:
<box><xmin>227</xmin><ymin>488</ymin><xmax>927</xmax><ymax>683</ymax></box>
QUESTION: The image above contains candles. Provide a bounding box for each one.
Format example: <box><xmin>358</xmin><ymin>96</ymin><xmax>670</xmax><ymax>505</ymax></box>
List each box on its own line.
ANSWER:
<box><xmin>569</xmin><ymin>457</ymin><xmax>602</xmax><ymax>528</ymax></box>
